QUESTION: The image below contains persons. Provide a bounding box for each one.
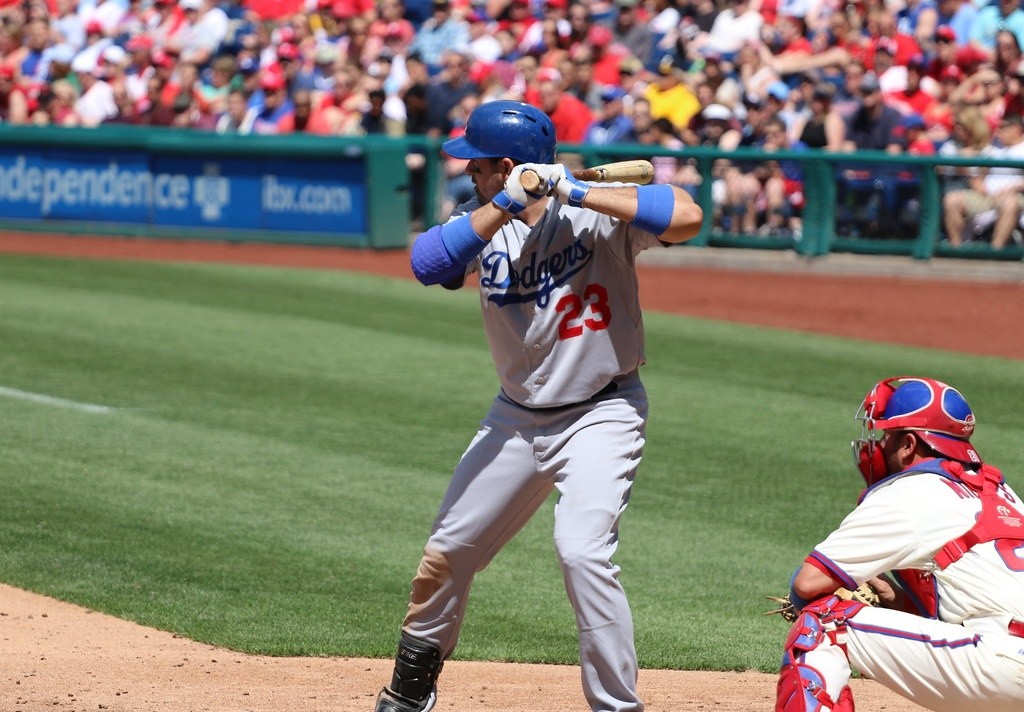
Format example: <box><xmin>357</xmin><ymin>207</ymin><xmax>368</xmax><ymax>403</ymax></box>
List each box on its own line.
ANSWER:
<box><xmin>0</xmin><ymin>0</ymin><xmax>1024</xmax><ymax>250</ymax></box>
<box><xmin>373</xmin><ymin>101</ymin><xmax>703</xmax><ymax>712</ymax></box>
<box><xmin>776</xmin><ymin>377</ymin><xmax>1024</xmax><ymax>712</ymax></box>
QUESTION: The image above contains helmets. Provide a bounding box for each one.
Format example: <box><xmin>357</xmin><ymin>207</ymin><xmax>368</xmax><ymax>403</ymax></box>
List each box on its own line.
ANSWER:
<box><xmin>441</xmin><ymin>99</ymin><xmax>558</xmax><ymax>165</ymax></box>
<box><xmin>851</xmin><ymin>375</ymin><xmax>984</xmax><ymax>488</ymax></box>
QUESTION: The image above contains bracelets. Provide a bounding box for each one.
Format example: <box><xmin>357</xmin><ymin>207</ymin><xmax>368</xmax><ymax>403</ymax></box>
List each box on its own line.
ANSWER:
<box><xmin>789</xmin><ymin>564</ymin><xmax>806</xmax><ymax>610</ymax></box>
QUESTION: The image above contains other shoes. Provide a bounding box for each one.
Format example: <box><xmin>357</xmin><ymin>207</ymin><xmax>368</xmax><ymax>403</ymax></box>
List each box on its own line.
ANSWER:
<box><xmin>374</xmin><ymin>630</ymin><xmax>444</xmax><ymax>712</ymax></box>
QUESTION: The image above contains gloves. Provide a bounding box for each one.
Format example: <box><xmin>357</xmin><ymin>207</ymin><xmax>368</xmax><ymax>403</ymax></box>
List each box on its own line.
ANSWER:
<box><xmin>491</xmin><ymin>162</ymin><xmax>592</xmax><ymax>217</ymax></box>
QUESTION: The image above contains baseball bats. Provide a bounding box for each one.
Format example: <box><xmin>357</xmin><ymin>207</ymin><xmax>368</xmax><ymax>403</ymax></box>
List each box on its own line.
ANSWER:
<box><xmin>520</xmin><ymin>159</ymin><xmax>655</xmax><ymax>191</ymax></box>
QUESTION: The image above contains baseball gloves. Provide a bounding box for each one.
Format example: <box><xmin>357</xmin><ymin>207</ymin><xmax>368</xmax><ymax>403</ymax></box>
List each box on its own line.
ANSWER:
<box><xmin>781</xmin><ymin>582</ymin><xmax>882</xmax><ymax>621</ymax></box>
